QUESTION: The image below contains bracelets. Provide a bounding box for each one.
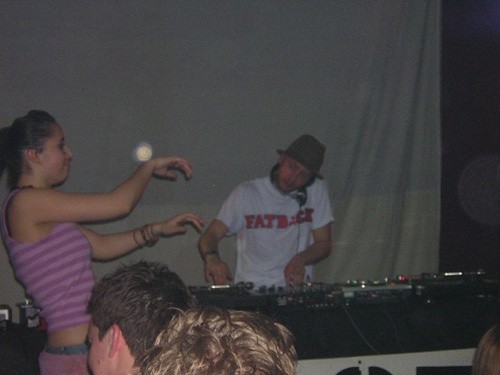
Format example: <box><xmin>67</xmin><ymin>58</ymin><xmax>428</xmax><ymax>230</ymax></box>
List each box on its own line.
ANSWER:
<box><xmin>202</xmin><ymin>251</ymin><xmax>219</xmax><ymax>260</ymax></box>
<box><xmin>133</xmin><ymin>222</ymin><xmax>160</xmax><ymax>249</ymax></box>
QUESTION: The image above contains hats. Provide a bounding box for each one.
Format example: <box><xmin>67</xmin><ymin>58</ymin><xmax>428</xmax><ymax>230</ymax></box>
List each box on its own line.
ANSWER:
<box><xmin>277</xmin><ymin>134</ymin><xmax>325</xmax><ymax>181</ymax></box>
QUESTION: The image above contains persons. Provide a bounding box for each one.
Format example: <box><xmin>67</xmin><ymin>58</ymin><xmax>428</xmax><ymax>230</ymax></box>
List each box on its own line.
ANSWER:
<box><xmin>1</xmin><ymin>110</ymin><xmax>204</xmax><ymax>375</ymax></box>
<box><xmin>470</xmin><ymin>324</ymin><xmax>500</xmax><ymax>375</ymax></box>
<box><xmin>84</xmin><ymin>257</ymin><xmax>297</xmax><ymax>375</ymax></box>
<box><xmin>198</xmin><ymin>133</ymin><xmax>336</xmax><ymax>289</ymax></box>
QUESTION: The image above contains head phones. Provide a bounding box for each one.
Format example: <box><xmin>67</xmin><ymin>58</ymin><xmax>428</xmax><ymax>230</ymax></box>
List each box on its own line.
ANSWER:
<box><xmin>270</xmin><ymin>163</ymin><xmax>315</xmax><ymax>206</ymax></box>
